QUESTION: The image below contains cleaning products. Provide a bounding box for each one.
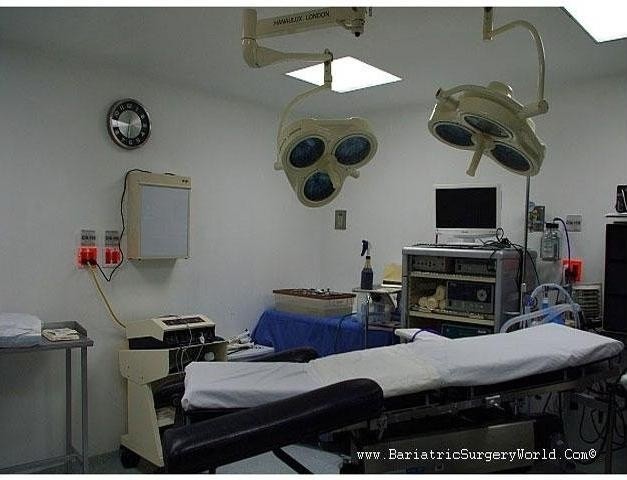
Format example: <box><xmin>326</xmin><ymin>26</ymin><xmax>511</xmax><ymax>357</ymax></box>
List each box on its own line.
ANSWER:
<box><xmin>360</xmin><ymin>238</ymin><xmax>373</xmax><ymax>289</ymax></box>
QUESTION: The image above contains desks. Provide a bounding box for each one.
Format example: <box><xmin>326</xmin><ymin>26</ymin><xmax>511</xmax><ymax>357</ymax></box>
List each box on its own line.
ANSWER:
<box><xmin>0</xmin><ymin>320</ymin><xmax>94</xmax><ymax>474</ymax></box>
<box><xmin>251</xmin><ymin>308</ymin><xmax>393</xmax><ymax>360</ymax></box>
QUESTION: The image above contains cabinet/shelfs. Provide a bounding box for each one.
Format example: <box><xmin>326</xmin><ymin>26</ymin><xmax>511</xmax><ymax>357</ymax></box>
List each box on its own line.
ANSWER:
<box><xmin>400</xmin><ymin>244</ymin><xmax>537</xmax><ymax>343</ymax></box>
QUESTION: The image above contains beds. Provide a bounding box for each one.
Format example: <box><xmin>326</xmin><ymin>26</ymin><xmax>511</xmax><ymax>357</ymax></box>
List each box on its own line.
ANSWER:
<box><xmin>162</xmin><ymin>323</ymin><xmax>625</xmax><ymax>474</ymax></box>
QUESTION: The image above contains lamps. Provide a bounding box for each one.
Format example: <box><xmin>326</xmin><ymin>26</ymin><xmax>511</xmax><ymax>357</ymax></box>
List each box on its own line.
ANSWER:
<box><xmin>241</xmin><ymin>7</ymin><xmax>377</xmax><ymax>207</ymax></box>
<box><xmin>427</xmin><ymin>8</ymin><xmax>549</xmax><ymax>178</ymax></box>
